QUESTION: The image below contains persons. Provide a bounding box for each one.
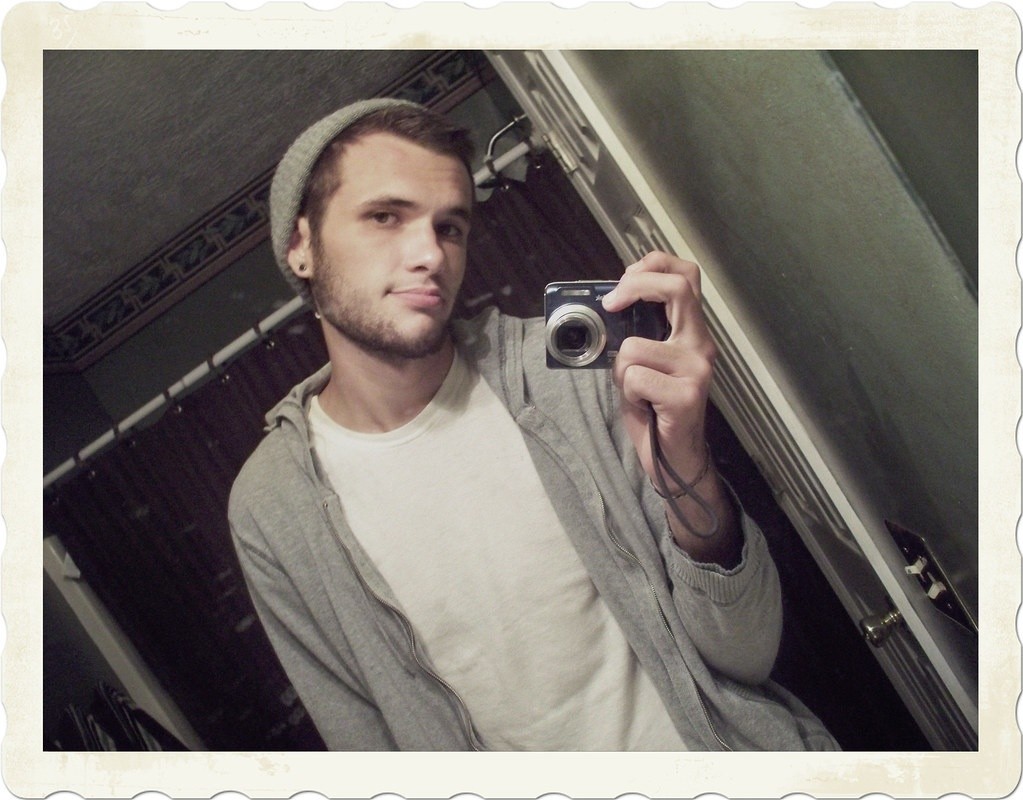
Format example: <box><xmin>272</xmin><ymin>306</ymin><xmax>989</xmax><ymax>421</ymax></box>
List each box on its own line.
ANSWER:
<box><xmin>225</xmin><ymin>96</ymin><xmax>841</xmax><ymax>752</ymax></box>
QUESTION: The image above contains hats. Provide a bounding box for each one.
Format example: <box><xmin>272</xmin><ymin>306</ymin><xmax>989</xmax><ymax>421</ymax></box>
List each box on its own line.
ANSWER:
<box><xmin>269</xmin><ymin>97</ymin><xmax>453</xmax><ymax>305</ymax></box>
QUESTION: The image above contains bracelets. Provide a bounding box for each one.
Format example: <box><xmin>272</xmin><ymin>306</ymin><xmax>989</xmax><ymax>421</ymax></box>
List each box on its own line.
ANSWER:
<box><xmin>649</xmin><ymin>440</ymin><xmax>711</xmax><ymax>499</ymax></box>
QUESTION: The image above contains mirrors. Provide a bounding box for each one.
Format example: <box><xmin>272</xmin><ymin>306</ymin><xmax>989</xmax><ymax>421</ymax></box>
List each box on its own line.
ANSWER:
<box><xmin>43</xmin><ymin>50</ymin><xmax>978</xmax><ymax>752</ymax></box>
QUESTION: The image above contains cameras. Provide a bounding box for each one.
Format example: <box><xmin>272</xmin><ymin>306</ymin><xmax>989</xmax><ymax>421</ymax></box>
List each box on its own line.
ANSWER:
<box><xmin>545</xmin><ymin>280</ymin><xmax>673</xmax><ymax>369</ymax></box>
